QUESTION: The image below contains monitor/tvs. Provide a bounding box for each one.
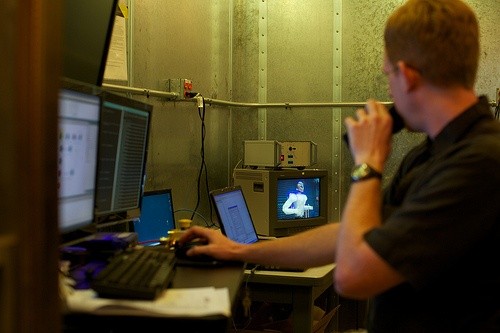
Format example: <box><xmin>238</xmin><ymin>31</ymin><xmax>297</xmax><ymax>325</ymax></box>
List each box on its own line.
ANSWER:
<box><xmin>128</xmin><ymin>189</ymin><xmax>176</xmax><ymax>248</ymax></box>
<box><xmin>234</xmin><ymin>169</ymin><xmax>328</xmax><ymax>237</ymax></box>
<box><xmin>58</xmin><ymin>75</ymin><xmax>153</xmax><ymax>243</ymax></box>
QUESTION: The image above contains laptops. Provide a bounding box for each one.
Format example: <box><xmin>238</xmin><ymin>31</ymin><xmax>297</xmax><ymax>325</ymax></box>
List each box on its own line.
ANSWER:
<box><xmin>209</xmin><ymin>185</ymin><xmax>305</xmax><ymax>272</ymax></box>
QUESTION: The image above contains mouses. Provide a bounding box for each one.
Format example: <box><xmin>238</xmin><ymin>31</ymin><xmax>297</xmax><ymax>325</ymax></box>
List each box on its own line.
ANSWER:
<box><xmin>174</xmin><ymin>243</ymin><xmax>216</xmax><ymax>264</ymax></box>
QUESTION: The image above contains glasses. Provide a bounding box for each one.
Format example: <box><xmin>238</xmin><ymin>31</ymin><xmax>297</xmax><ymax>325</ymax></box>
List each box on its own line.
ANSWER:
<box><xmin>376</xmin><ymin>69</ymin><xmax>396</xmax><ymax>88</ymax></box>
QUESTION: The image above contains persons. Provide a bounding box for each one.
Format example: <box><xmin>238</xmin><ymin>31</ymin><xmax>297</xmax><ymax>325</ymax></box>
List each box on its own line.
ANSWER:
<box><xmin>178</xmin><ymin>0</ymin><xmax>499</xmax><ymax>333</ymax></box>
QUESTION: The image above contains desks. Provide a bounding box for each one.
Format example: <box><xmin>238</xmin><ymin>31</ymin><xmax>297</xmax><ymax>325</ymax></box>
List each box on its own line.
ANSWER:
<box><xmin>243</xmin><ymin>263</ymin><xmax>337</xmax><ymax>333</ymax></box>
<box><xmin>59</xmin><ymin>262</ymin><xmax>245</xmax><ymax>333</ymax></box>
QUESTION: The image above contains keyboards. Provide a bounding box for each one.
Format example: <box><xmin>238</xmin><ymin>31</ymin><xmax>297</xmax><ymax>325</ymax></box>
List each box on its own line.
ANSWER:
<box><xmin>90</xmin><ymin>247</ymin><xmax>177</xmax><ymax>300</ymax></box>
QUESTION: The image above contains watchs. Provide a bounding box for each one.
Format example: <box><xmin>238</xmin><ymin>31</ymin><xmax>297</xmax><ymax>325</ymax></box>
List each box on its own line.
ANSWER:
<box><xmin>349</xmin><ymin>163</ymin><xmax>384</xmax><ymax>183</ymax></box>
